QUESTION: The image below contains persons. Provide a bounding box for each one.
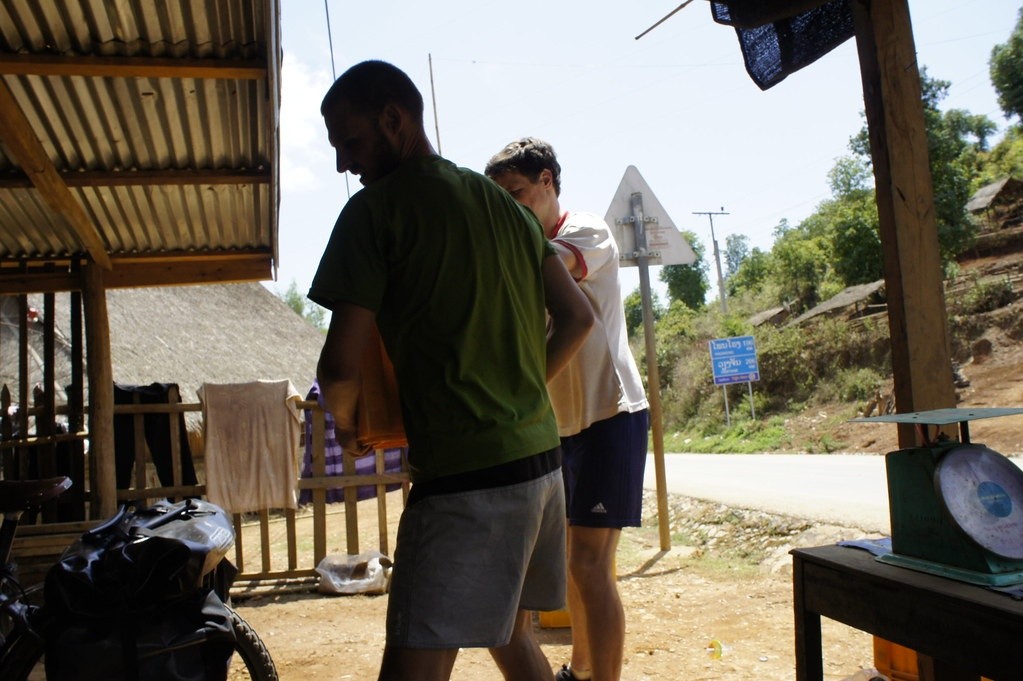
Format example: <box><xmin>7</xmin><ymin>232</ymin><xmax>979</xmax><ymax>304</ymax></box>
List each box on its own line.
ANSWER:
<box><xmin>306</xmin><ymin>60</ymin><xmax>597</xmax><ymax>680</ymax></box>
<box><xmin>482</xmin><ymin>138</ymin><xmax>652</xmax><ymax>681</ymax></box>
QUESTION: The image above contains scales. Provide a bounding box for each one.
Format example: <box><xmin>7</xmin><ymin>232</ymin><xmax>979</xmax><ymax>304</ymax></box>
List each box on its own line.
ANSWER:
<box><xmin>844</xmin><ymin>400</ymin><xmax>1022</xmax><ymax>587</ymax></box>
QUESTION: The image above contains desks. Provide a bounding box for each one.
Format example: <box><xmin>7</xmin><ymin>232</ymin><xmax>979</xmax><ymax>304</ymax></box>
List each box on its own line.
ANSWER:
<box><xmin>789</xmin><ymin>539</ymin><xmax>1023</xmax><ymax>681</ymax></box>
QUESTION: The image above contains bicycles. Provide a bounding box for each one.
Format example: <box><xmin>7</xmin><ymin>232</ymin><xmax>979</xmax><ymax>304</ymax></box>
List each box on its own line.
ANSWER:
<box><xmin>0</xmin><ymin>474</ymin><xmax>279</xmax><ymax>681</ymax></box>
